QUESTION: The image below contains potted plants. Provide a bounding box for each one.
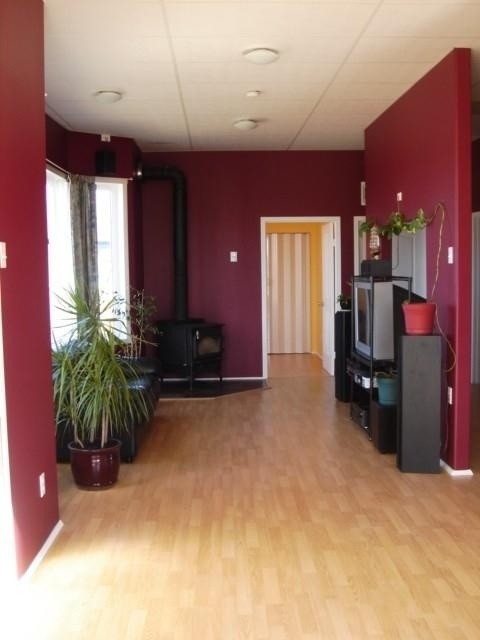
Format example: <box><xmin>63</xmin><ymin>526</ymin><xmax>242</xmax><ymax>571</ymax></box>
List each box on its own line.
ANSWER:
<box><xmin>337</xmin><ymin>294</ymin><xmax>351</xmax><ymax>309</ymax></box>
<box><xmin>51</xmin><ymin>280</ymin><xmax>157</xmax><ymax>490</ymax></box>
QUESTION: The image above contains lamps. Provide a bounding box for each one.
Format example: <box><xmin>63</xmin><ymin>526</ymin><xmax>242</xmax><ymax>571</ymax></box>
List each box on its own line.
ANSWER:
<box><xmin>244</xmin><ymin>48</ymin><xmax>278</xmax><ymax>64</ymax></box>
<box><xmin>235</xmin><ymin>120</ymin><xmax>257</xmax><ymax>131</ymax></box>
<box><xmin>93</xmin><ymin>91</ymin><xmax>121</xmax><ymax>103</ymax></box>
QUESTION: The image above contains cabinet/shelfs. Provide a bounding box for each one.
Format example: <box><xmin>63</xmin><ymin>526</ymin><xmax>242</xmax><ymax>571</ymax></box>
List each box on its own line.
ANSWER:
<box><xmin>350</xmin><ymin>276</ymin><xmax>412</xmax><ymax>441</ymax></box>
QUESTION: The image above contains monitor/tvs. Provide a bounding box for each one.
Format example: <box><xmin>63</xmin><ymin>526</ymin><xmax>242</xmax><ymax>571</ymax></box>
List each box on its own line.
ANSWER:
<box><xmin>353</xmin><ymin>280</ymin><xmax>427</xmax><ymax>360</ymax></box>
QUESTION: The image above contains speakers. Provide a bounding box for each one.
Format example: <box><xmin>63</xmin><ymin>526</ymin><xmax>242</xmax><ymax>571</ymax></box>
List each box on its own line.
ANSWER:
<box><xmin>96</xmin><ymin>150</ymin><xmax>115</xmax><ymax>176</ymax></box>
<box><xmin>334</xmin><ymin>310</ymin><xmax>352</xmax><ymax>402</ymax></box>
<box><xmin>396</xmin><ymin>335</ymin><xmax>441</xmax><ymax>473</ymax></box>
<box><xmin>360</xmin><ymin>260</ymin><xmax>392</xmax><ymax>281</ymax></box>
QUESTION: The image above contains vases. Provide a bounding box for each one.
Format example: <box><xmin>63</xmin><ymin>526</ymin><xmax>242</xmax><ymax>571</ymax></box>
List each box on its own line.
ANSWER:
<box><xmin>403</xmin><ymin>304</ymin><xmax>435</xmax><ymax>335</ymax></box>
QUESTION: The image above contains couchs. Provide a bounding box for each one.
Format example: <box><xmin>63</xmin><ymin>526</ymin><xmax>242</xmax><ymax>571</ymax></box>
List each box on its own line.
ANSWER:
<box><xmin>57</xmin><ymin>358</ymin><xmax>161</xmax><ymax>463</ymax></box>
